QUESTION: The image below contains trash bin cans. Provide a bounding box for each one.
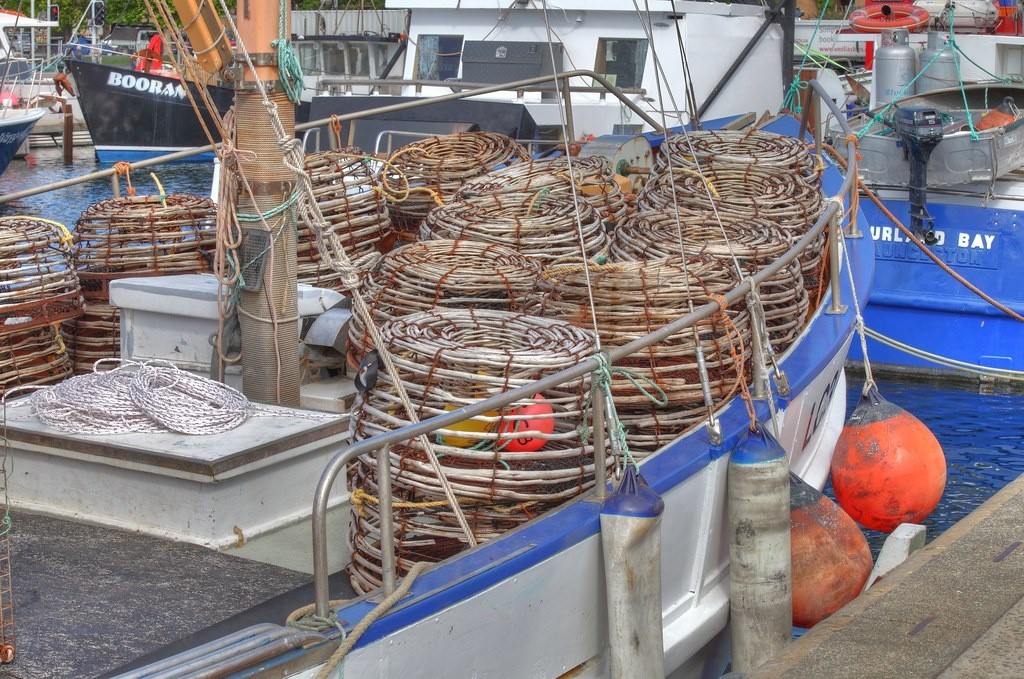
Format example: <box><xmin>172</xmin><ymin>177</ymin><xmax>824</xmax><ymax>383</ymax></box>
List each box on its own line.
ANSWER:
<box><xmin>107</xmin><ymin>273</ymin><xmax>328</xmax><ymax>397</ymax></box>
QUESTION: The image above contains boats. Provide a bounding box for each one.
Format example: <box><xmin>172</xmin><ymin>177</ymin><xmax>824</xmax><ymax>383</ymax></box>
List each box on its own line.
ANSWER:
<box><xmin>1</xmin><ymin>0</ymin><xmax>1023</xmax><ymax>679</ymax></box>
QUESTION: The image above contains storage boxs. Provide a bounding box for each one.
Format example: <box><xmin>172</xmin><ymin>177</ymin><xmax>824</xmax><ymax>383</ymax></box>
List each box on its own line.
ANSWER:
<box><xmin>106</xmin><ymin>274</ymin><xmax>345</xmax><ymax>399</ymax></box>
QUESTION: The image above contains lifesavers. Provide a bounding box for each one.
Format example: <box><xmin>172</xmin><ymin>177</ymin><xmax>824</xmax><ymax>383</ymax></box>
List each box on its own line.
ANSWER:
<box><xmin>849</xmin><ymin>3</ymin><xmax>929</xmax><ymax>33</ymax></box>
